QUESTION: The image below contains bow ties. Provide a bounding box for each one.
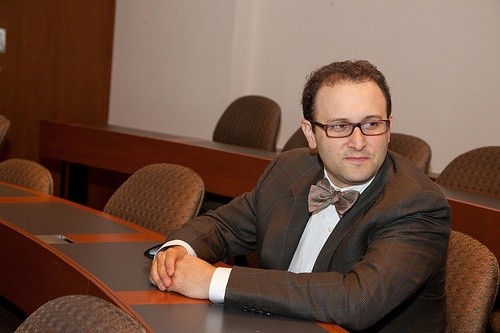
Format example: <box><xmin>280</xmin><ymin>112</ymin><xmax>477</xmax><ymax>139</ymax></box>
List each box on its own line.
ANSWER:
<box><xmin>307</xmin><ymin>178</ymin><xmax>360</xmax><ymax>218</ymax></box>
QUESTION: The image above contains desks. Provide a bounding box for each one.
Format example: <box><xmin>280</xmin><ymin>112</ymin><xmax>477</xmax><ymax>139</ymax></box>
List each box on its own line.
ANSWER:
<box><xmin>38</xmin><ymin>120</ymin><xmax>500</xmax><ymax>309</ymax></box>
<box><xmin>0</xmin><ymin>180</ymin><xmax>348</xmax><ymax>333</ymax></box>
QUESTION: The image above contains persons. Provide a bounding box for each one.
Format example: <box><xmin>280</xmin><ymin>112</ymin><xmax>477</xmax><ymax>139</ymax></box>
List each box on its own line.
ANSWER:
<box><xmin>143</xmin><ymin>59</ymin><xmax>450</xmax><ymax>333</ymax></box>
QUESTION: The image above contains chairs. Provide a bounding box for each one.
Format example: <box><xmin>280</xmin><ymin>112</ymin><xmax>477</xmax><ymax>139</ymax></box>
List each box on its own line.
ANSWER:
<box><xmin>103</xmin><ymin>163</ymin><xmax>205</xmax><ymax>237</ymax></box>
<box><xmin>0</xmin><ymin>159</ymin><xmax>54</xmax><ymax>196</ymax></box>
<box><xmin>212</xmin><ymin>95</ymin><xmax>431</xmax><ymax>176</ymax></box>
<box><xmin>14</xmin><ymin>295</ymin><xmax>148</xmax><ymax>332</ymax></box>
<box><xmin>435</xmin><ymin>146</ymin><xmax>500</xmax><ymax>200</ymax></box>
<box><xmin>443</xmin><ymin>230</ymin><xmax>500</xmax><ymax>333</ymax></box>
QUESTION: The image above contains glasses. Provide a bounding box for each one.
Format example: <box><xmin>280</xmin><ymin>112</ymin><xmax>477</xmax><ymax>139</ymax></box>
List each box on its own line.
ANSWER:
<box><xmin>309</xmin><ymin>117</ymin><xmax>390</xmax><ymax>138</ymax></box>
